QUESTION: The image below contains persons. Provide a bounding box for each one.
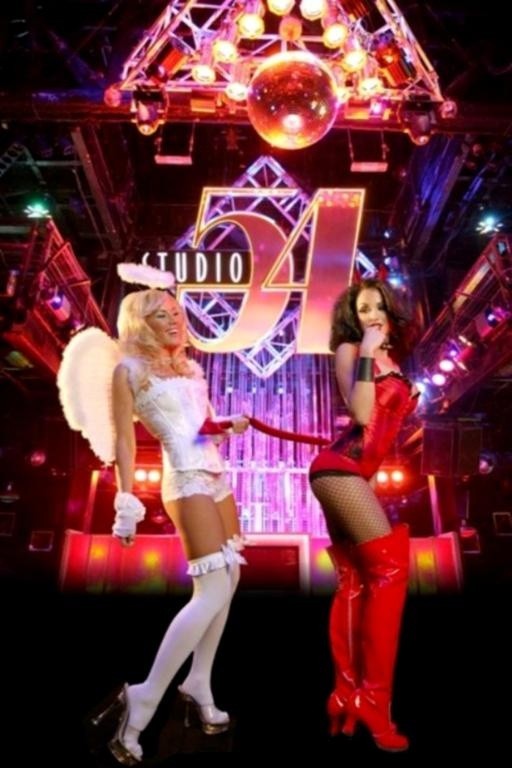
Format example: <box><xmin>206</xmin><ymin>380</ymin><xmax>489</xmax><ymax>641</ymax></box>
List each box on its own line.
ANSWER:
<box><xmin>88</xmin><ymin>287</ymin><xmax>254</xmax><ymax>764</ymax></box>
<box><xmin>303</xmin><ymin>277</ymin><xmax>425</xmax><ymax>757</ymax></box>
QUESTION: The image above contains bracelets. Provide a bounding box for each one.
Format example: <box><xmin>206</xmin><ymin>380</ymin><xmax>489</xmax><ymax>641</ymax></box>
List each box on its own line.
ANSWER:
<box><xmin>351</xmin><ymin>356</ymin><xmax>377</xmax><ymax>384</ymax></box>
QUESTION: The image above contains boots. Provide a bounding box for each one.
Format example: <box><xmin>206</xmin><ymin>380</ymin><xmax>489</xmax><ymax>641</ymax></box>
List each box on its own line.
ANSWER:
<box><xmin>325</xmin><ymin>522</ymin><xmax>410</xmax><ymax>753</ymax></box>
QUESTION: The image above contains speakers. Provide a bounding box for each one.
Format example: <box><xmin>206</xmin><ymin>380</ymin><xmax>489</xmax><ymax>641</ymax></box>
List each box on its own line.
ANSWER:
<box><xmin>420</xmin><ymin>419</ymin><xmax>455</xmax><ymax>478</ymax></box>
<box><xmin>456</xmin><ymin>422</ymin><xmax>482</xmax><ymax>474</ymax></box>
<box><xmin>232</xmin><ymin>532</ymin><xmax>310</xmax><ymax>594</ymax></box>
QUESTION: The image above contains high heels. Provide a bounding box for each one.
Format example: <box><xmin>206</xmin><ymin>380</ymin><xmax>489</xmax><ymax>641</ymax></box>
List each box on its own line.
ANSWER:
<box><xmin>88</xmin><ymin>681</ymin><xmax>146</xmax><ymax>766</ymax></box>
<box><xmin>177</xmin><ymin>685</ymin><xmax>230</xmax><ymax>735</ymax></box>
